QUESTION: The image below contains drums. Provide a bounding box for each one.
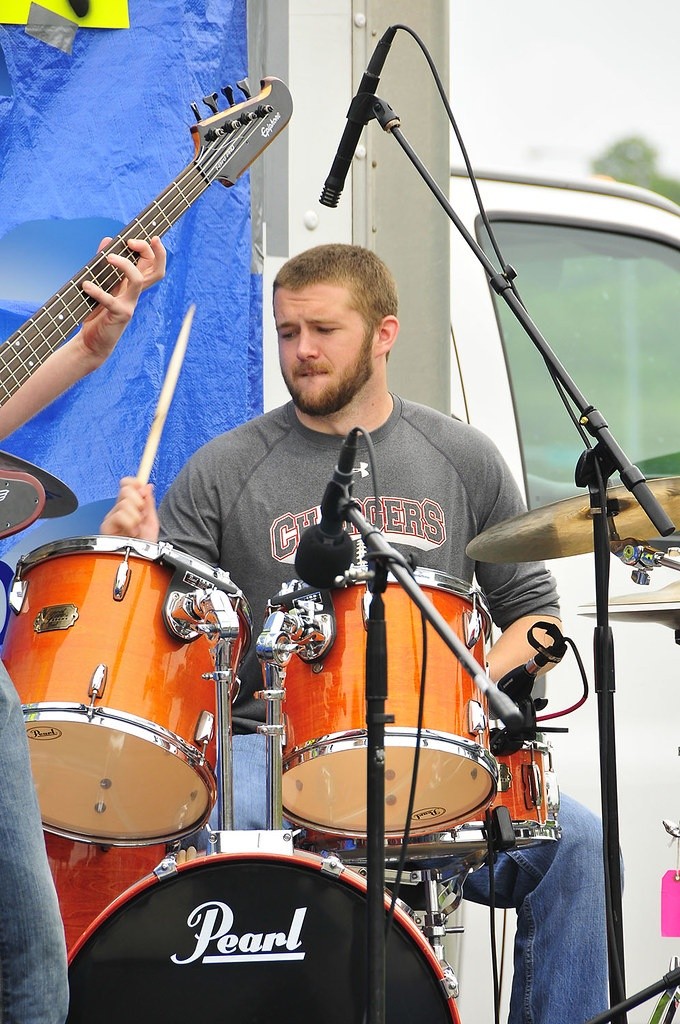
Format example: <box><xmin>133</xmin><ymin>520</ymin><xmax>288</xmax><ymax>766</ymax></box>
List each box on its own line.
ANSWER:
<box><xmin>260</xmin><ymin>559</ymin><xmax>500</xmax><ymax>843</ymax></box>
<box><xmin>285</xmin><ymin>737</ymin><xmax>564</xmax><ymax>872</ymax></box>
<box><xmin>67</xmin><ymin>843</ymin><xmax>463</xmax><ymax>1024</ymax></box>
<box><xmin>0</xmin><ymin>532</ymin><xmax>256</xmax><ymax>851</ymax></box>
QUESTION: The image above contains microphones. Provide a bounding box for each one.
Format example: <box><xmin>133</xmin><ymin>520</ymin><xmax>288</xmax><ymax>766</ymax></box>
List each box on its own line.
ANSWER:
<box><xmin>319</xmin><ymin>26</ymin><xmax>398</xmax><ymax>208</ymax></box>
<box><xmin>294</xmin><ymin>428</ymin><xmax>360</xmax><ymax>589</ymax></box>
<box><xmin>494</xmin><ymin>646</ymin><xmax>556</xmax><ymax>704</ymax></box>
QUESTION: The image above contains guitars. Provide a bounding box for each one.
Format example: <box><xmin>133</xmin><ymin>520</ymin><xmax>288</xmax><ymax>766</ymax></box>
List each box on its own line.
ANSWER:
<box><xmin>0</xmin><ymin>74</ymin><xmax>295</xmax><ymax>416</ymax></box>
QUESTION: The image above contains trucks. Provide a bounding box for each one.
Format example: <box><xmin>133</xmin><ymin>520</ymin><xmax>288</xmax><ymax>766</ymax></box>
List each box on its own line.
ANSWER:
<box><xmin>1</xmin><ymin>3</ymin><xmax>680</xmax><ymax>1024</ymax></box>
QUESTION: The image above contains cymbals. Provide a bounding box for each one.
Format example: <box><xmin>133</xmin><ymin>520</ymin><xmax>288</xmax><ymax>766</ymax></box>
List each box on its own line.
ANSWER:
<box><xmin>464</xmin><ymin>474</ymin><xmax>680</xmax><ymax>566</ymax></box>
<box><xmin>0</xmin><ymin>449</ymin><xmax>81</xmax><ymax>519</ymax></box>
<box><xmin>576</xmin><ymin>579</ymin><xmax>680</xmax><ymax>629</ymax></box>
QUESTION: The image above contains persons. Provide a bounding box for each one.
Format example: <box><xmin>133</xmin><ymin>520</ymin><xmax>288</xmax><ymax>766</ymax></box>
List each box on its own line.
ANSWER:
<box><xmin>100</xmin><ymin>242</ymin><xmax>626</xmax><ymax>1023</ymax></box>
<box><xmin>0</xmin><ymin>235</ymin><xmax>167</xmax><ymax>1024</ymax></box>
